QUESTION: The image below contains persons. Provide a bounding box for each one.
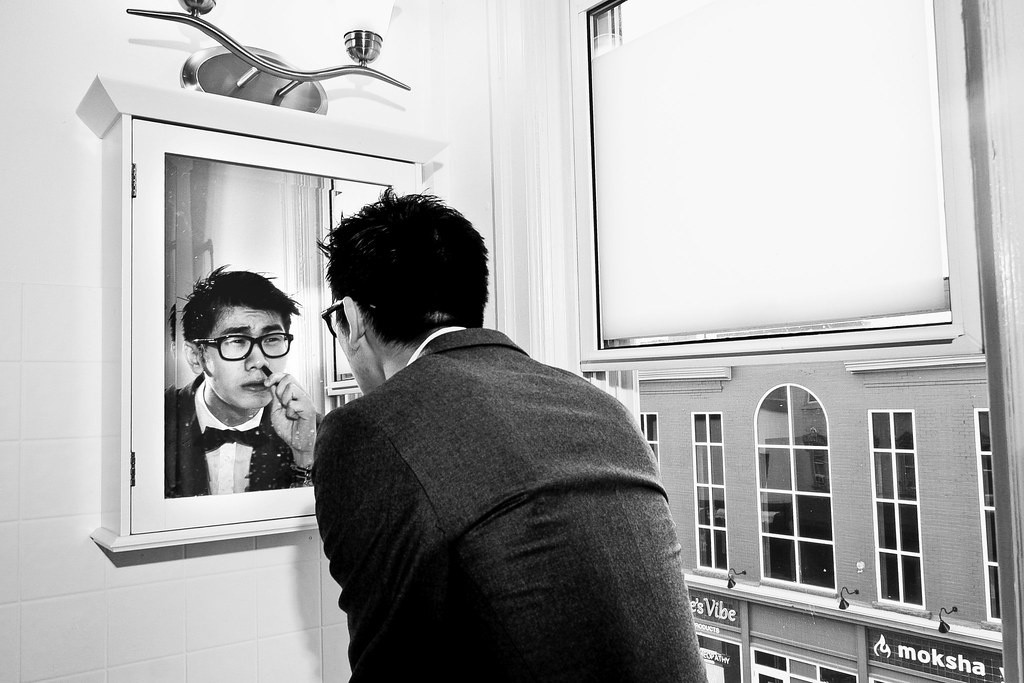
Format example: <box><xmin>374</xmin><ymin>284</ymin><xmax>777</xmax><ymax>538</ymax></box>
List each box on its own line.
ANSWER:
<box><xmin>311</xmin><ymin>184</ymin><xmax>709</xmax><ymax>682</ymax></box>
<box><xmin>165</xmin><ymin>265</ymin><xmax>322</xmax><ymax>497</ymax></box>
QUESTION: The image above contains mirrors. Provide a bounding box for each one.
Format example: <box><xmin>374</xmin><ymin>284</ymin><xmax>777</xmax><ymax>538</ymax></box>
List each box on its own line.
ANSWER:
<box><xmin>163</xmin><ymin>152</ymin><xmax>395</xmax><ymax>501</ymax></box>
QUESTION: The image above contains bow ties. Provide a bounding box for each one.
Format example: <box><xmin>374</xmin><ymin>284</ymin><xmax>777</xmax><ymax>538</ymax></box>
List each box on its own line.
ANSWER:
<box><xmin>200</xmin><ymin>425</ymin><xmax>268</xmax><ymax>453</ymax></box>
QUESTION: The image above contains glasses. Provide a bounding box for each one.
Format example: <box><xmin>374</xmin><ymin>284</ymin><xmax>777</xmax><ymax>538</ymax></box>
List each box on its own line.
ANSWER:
<box><xmin>320</xmin><ymin>299</ymin><xmax>380</xmax><ymax>336</ymax></box>
<box><xmin>192</xmin><ymin>333</ymin><xmax>294</xmax><ymax>361</ymax></box>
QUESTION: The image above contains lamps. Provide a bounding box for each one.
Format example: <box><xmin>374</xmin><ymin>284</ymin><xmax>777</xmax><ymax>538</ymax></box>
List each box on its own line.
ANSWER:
<box><xmin>127</xmin><ymin>1</ymin><xmax>412</xmax><ymax>113</ymax></box>
